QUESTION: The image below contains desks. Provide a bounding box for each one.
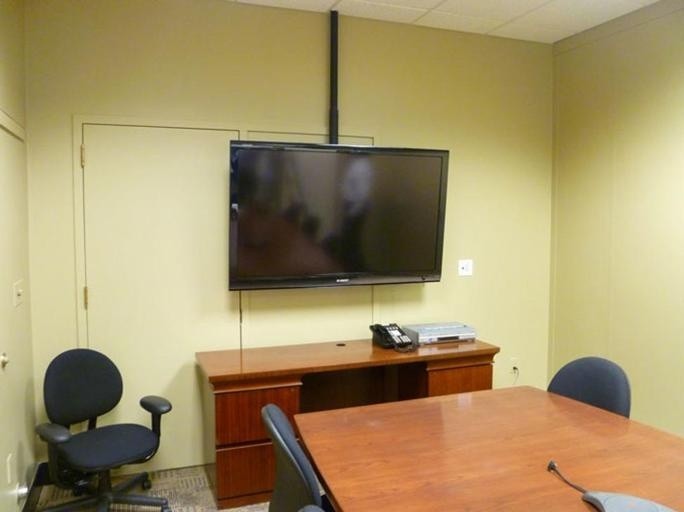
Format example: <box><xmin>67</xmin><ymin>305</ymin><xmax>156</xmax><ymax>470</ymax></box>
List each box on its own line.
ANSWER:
<box><xmin>195</xmin><ymin>337</ymin><xmax>500</xmax><ymax>510</ymax></box>
<box><xmin>294</xmin><ymin>384</ymin><xmax>684</xmax><ymax>512</ymax></box>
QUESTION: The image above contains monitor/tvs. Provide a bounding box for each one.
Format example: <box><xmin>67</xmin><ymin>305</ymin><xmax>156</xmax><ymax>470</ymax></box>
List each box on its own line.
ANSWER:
<box><xmin>228</xmin><ymin>139</ymin><xmax>449</xmax><ymax>291</ymax></box>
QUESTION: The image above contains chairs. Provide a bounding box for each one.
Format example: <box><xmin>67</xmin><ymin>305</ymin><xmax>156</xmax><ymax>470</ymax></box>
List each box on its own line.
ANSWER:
<box><xmin>547</xmin><ymin>357</ymin><xmax>631</xmax><ymax>418</ymax></box>
<box><xmin>34</xmin><ymin>349</ymin><xmax>172</xmax><ymax>512</ymax></box>
<box><xmin>261</xmin><ymin>404</ymin><xmax>335</xmax><ymax>512</ymax></box>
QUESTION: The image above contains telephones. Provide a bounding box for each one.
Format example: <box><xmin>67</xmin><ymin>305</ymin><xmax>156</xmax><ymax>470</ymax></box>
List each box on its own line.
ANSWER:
<box><xmin>369</xmin><ymin>323</ymin><xmax>412</xmax><ymax>349</ymax></box>
